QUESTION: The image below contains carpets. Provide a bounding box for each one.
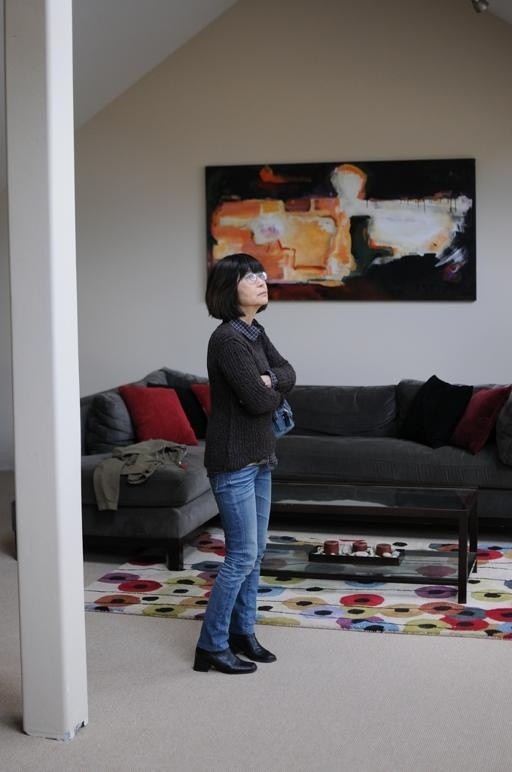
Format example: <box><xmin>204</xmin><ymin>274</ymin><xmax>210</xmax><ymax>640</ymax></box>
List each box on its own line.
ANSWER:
<box><xmin>84</xmin><ymin>532</ymin><xmax>510</xmax><ymax>643</ymax></box>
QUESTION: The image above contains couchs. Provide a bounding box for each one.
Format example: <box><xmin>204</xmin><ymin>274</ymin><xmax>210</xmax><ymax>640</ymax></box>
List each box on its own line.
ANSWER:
<box><xmin>81</xmin><ymin>363</ymin><xmax>510</xmax><ymax>572</ymax></box>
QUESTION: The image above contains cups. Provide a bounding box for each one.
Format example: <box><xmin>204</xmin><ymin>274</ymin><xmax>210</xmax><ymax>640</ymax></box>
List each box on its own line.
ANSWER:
<box><xmin>375</xmin><ymin>543</ymin><xmax>392</xmax><ymax>557</ymax></box>
<box><xmin>352</xmin><ymin>541</ymin><xmax>367</xmax><ymax>551</ymax></box>
<box><xmin>324</xmin><ymin>540</ymin><xmax>338</xmax><ymax>553</ymax></box>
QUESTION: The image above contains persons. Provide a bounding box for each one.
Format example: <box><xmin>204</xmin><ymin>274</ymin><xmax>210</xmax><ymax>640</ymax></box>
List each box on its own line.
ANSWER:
<box><xmin>192</xmin><ymin>252</ymin><xmax>297</xmax><ymax>674</ymax></box>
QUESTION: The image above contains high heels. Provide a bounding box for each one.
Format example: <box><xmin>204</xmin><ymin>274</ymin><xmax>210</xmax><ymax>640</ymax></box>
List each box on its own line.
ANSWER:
<box><xmin>193</xmin><ymin>644</ymin><xmax>257</xmax><ymax>674</ymax></box>
<box><xmin>228</xmin><ymin>633</ymin><xmax>276</xmax><ymax>663</ymax></box>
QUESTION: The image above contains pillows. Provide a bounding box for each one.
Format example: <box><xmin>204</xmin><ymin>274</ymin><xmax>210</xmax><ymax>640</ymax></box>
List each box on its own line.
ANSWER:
<box><xmin>117</xmin><ymin>383</ymin><xmax>200</xmax><ymax>448</ymax></box>
<box><xmin>189</xmin><ymin>381</ymin><xmax>215</xmax><ymax>415</ymax></box>
<box><xmin>147</xmin><ymin>381</ymin><xmax>211</xmax><ymax>441</ymax></box>
<box><xmin>452</xmin><ymin>384</ymin><xmax>511</xmax><ymax>455</ymax></box>
<box><xmin>85</xmin><ymin>394</ymin><xmax>131</xmax><ymax>456</ymax></box>
<box><xmin>388</xmin><ymin>374</ymin><xmax>473</xmax><ymax>449</ymax></box>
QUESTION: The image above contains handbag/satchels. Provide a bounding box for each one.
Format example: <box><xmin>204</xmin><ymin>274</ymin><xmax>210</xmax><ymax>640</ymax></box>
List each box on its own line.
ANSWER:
<box><xmin>272</xmin><ymin>398</ymin><xmax>296</xmax><ymax>440</ymax></box>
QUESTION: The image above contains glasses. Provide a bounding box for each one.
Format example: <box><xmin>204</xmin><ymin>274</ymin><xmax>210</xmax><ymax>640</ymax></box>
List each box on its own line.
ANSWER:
<box><xmin>244</xmin><ymin>272</ymin><xmax>267</xmax><ymax>282</ymax></box>
